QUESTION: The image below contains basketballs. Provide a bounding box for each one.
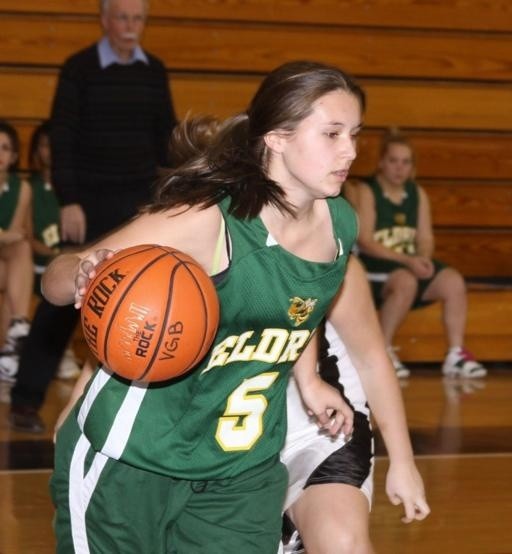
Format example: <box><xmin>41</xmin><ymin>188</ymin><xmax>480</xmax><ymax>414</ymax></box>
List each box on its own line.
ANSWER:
<box><xmin>81</xmin><ymin>245</ymin><xmax>219</xmax><ymax>382</ymax></box>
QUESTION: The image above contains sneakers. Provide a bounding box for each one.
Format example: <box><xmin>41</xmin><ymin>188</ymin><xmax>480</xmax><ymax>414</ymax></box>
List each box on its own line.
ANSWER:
<box><xmin>443</xmin><ymin>352</ymin><xmax>487</xmax><ymax>378</ymax></box>
<box><xmin>387</xmin><ymin>350</ymin><xmax>409</xmax><ymax>377</ymax></box>
<box><xmin>1</xmin><ymin>319</ymin><xmax>82</xmax><ymax>434</ymax></box>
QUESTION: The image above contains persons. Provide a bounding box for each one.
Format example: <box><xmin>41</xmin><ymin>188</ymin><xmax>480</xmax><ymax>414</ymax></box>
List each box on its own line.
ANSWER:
<box><xmin>26</xmin><ymin>119</ymin><xmax>82</xmax><ymax>378</ymax></box>
<box><xmin>348</xmin><ymin>132</ymin><xmax>488</xmax><ymax>380</ymax></box>
<box><xmin>1</xmin><ymin>116</ymin><xmax>40</xmax><ymax>381</ymax></box>
<box><xmin>9</xmin><ymin>1</ymin><xmax>185</xmax><ymax>432</ymax></box>
<box><xmin>155</xmin><ymin>101</ymin><xmax>431</xmax><ymax>554</ymax></box>
<box><xmin>38</xmin><ymin>60</ymin><xmax>368</xmax><ymax>553</ymax></box>
